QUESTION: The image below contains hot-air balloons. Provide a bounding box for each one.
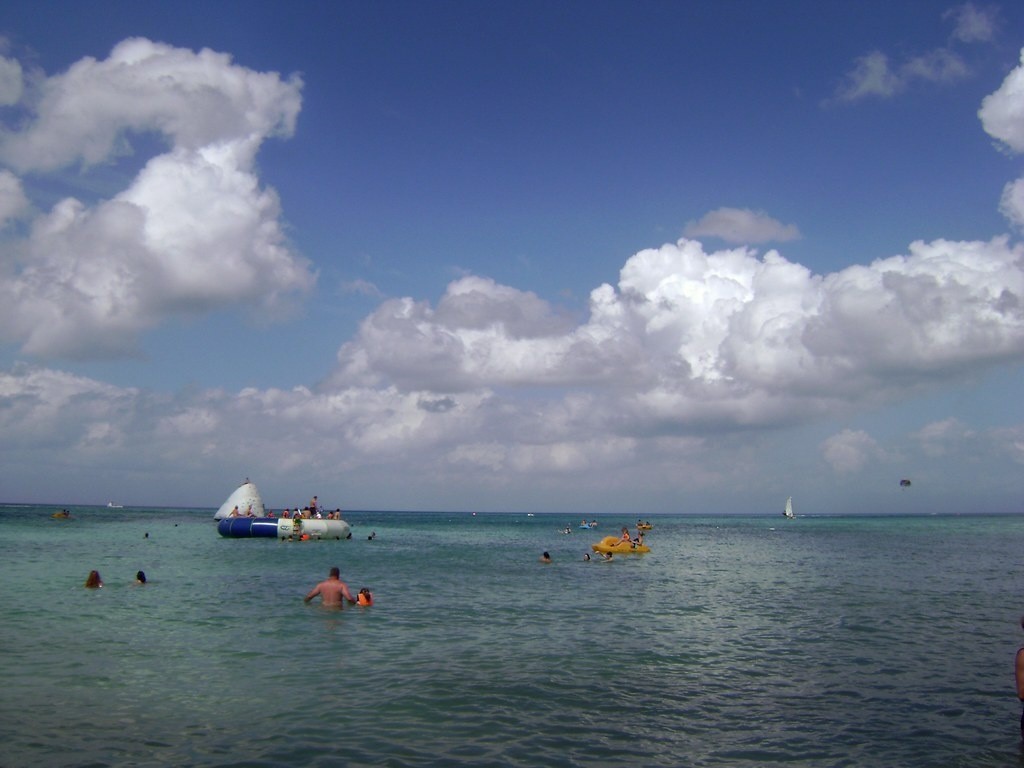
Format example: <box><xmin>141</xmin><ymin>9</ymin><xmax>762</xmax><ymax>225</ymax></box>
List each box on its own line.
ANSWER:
<box><xmin>899</xmin><ymin>478</ymin><xmax>911</xmax><ymax>493</ymax></box>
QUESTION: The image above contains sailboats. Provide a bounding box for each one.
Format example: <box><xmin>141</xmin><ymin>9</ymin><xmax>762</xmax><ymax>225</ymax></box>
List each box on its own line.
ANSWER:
<box><xmin>782</xmin><ymin>496</ymin><xmax>797</xmax><ymax>519</ymax></box>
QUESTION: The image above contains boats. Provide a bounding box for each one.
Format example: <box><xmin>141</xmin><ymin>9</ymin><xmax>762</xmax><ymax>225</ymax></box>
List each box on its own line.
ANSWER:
<box><xmin>216</xmin><ymin>516</ymin><xmax>351</xmax><ymax>541</ymax></box>
<box><xmin>636</xmin><ymin>525</ymin><xmax>652</xmax><ymax>531</ymax></box>
<box><xmin>105</xmin><ymin>500</ymin><xmax>125</xmax><ymax>509</ymax></box>
<box><xmin>579</xmin><ymin>524</ymin><xmax>593</xmax><ymax>530</ymax></box>
<box><xmin>591</xmin><ymin>535</ymin><xmax>651</xmax><ymax>554</ymax></box>
<box><xmin>52</xmin><ymin>512</ymin><xmax>78</xmax><ymax>520</ymax></box>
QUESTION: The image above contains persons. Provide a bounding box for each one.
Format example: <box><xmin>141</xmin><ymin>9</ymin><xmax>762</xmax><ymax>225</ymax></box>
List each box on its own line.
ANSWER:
<box><xmin>541</xmin><ymin>552</ymin><xmax>551</xmax><ymax>562</ymax></box>
<box><xmin>304</xmin><ymin>567</ymin><xmax>355</xmax><ymax>608</ymax></box>
<box><xmin>584</xmin><ymin>553</ymin><xmax>591</xmax><ymax>561</ymax></box>
<box><xmin>611</xmin><ymin>527</ymin><xmax>645</xmax><ymax>548</ymax></box>
<box><xmin>86</xmin><ymin>570</ymin><xmax>103</xmax><ymax>589</ymax></box>
<box><xmin>281</xmin><ymin>532</ymin><xmax>310</xmax><ymax>542</ymax></box>
<box><xmin>268</xmin><ymin>506</ymin><xmax>311</xmax><ymax>518</ymax></box>
<box><xmin>638</xmin><ymin>520</ymin><xmax>650</xmax><ymax>526</ymax></box>
<box><xmin>327</xmin><ymin>508</ymin><xmax>340</xmax><ymax>520</ymax></box>
<box><xmin>63</xmin><ymin>509</ymin><xmax>70</xmax><ymax>517</ymax></box>
<box><xmin>581</xmin><ymin>520</ymin><xmax>586</xmax><ymax>526</ymax></box>
<box><xmin>136</xmin><ymin>570</ymin><xmax>146</xmax><ymax>584</ymax></box>
<box><xmin>310</xmin><ymin>496</ymin><xmax>318</xmax><ymax>519</ymax></box>
<box><xmin>595</xmin><ymin>551</ymin><xmax>614</xmax><ymax>562</ymax></box>
<box><xmin>346</xmin><ymin>532</ymin><xmax>377</xmax><ymax>540</ymax></box>
<box><xmin>315</xmin><ymin>505</ymin><xmax>325</xmax><ymax>519</ymax></box>
<box><xmin>246</xmin><ymin>505</ymin><xmax>254</xmax><ymax>517</ymax></box>
<box><xmin>356</xmin><ymin>588</ymin><xmax>372</xmax><ymax>606</ymax></box>
<box><xmin>228</xmin><ymin>505</ymin><xmax>242</xmax><ymax>516</ymax></box>
<box><xmin>590</xmin><ymin>520</ymin><xmax>597</xmax><ymax>527</ymax></box>
